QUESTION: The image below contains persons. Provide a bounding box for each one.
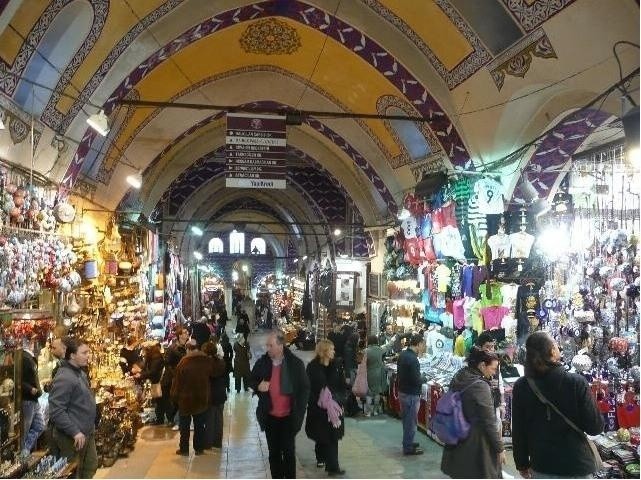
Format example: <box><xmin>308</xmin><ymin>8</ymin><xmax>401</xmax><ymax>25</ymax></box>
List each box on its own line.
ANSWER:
<box><xmin>38</xmin><ymin>336</ymin><xmax>67</xmax><ymax>451</ymax></box>
<box><xmin>348</xmin><ymin>312</ymin><xmax>368</xmax><ymax>414</ymax></box>
<box><xmin>397</xmin><ymin>334</ymin><xmax>430</xmax><ymax>455</ymax></box>
<box><xmin>165</xmin><ymin>325</ymin><xmax>195</xmax><ymax>431</ymax></box>
<box><xmin>186</xmin><ymin>284</ymin><xmax>275</xmax><ymax>338</ymax></box>
<box><xmin>326</xmin><ymin>317</ymin><xmax>348</xmax><ymax>339</ymax></box>
<box><xmin>288</xmin><ymin>325</ymin><xmax>316</xmax><ymax>350</ymax></box>
<box><xmin>200</xmin><ymin>342</ymin><xmax>229</xmax><ymax>450</ymax></box>
<box><xmin>475</xmin><ymin>333</ymin><xmax>507</xmax><ymax>438</ymax></box>
<box><xmin>365</xmin><ymin>333</ymin><xmax>397</xmax><ymax>417</ymax></box>
<box><xmin>176</xmin><ymin>339</ymin><xmax>226</xmax><ymax>456</ymax></box>
<box><xmin>119</xmin><ymin>332</ymin><xmax>144</xmax><ymax>414</ymax></box>
<box><xmin>306</xmin><ymin>340</ymin><xmax>348</xmax><ymax>476</ymax></box>
<box><xmin>336</xmin><ymin>340</ymin><xmax>348</xmax><ymax>376</ymax></box>
<box><xmin>249</xmin><ymin>331</ymin><xmax>311</xmax><ymax>478</ymax></box>
<box><xmin>281</xmin><ymin>305</ymin><xmax>291</xmax><ymax>325</ymax></box>
<box><xmin>512</xmin><ymin>332</ymin><xmax>606</xmax><ymax>479</ymax></box>
<box><xmin>134</xmin><ymin>340</ymin><xmax>166</xmax><ymax>425</ymax></box>
<box><xmin>441</xmin><ymin>346</ymin><xmax>509</xmax><ymax>479</ymax></box>
<box><xmin>235</xmin><ymin>333</ymin><xmax>253</xmax><ymax>393</ymax></box>
<box><xmin>221</xmin><ymin>334</ymin><xmax>234</xmax><ymax>393</ymax></box>
<box><xmin>22</xmin><ymin>332</ymin><xmax>46</xmax><ymax>457</ymax></box>
<box><xmin>380</xmin><ymin>323</ymin><xmax>419</xmax><ymax>359</ymax></box>
<box><xmin>214</xmin><ymin>334</ymin><xmax>225</xmax><ymax>360</ymax></box>
<box><xmin>49</xmin><ymin>339</ymin><xmax>99</xmax><ymax>479</ymax></box>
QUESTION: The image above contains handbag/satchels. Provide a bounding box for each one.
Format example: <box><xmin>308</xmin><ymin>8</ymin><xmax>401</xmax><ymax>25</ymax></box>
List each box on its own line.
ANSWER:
<box><xmin>587</xmin><ymin>438</ymin><xmax>602</xmax><ymax>472</ymax></box>
<box><xmin>151</xmin><ymin>383</ymin><xmax>162</xmax><ymax>398</ymax></box>
<box><xmin>351</xmin><ymin>354</ymin><xmax>369</xmax><ymax>397</ymax></box>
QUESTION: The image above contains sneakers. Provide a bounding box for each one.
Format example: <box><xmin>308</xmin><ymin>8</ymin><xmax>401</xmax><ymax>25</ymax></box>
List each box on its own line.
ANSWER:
<box><xmin>317</xmin><ymin>459</ymin><xmax>324</xmax><ymax>468</ymax></box>
<box><xmin>171</xmin><ymin>425</ymin><xmax>179</xmax><ymax>430</ymax></box>
<box><xmin>176</xmin><ymin>450</ymin><xmax>189</xmax><ymax>456</ymax></box>
<box><xmin>195</xmin><ymin>448</ymin><xmax>204</xmax><ymax>455</ymax></box>
<box><xmin>328</xmin><ymin>470</ymin><xmax>345</xmax><ymax>475</ymax></box>
<box><xmin>408</xmin><ymin>448</ymin><xmax>423</xmax><ymax>455</ymax></box>
<box><xmin>413</xmin><ymin>441</ymin><xmax>419</xmax><ymax>446</ymax></box>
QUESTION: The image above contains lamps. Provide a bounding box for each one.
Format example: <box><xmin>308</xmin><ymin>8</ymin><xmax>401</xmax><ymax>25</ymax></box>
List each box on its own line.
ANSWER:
<box><xmin>0</xmin><ymin>69</ymin><xmax>112</xmax><ymax>137</ymax></box>
<box><xmin>517</xmin><ymin>169</ymin><xmax>639</xmax><ymax>217</ymax></box>
<box><xmin>89</xmin><ymin>148</ymin><xmax>143</xmax><ymax>189</ymax></box>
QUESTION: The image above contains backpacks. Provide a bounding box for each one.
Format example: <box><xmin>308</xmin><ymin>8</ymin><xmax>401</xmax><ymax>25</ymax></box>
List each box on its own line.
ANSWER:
<box><xmin>433</xmin><ymin>379</ymin><xmax>481</xmax><ymax>445</ymax></box>
